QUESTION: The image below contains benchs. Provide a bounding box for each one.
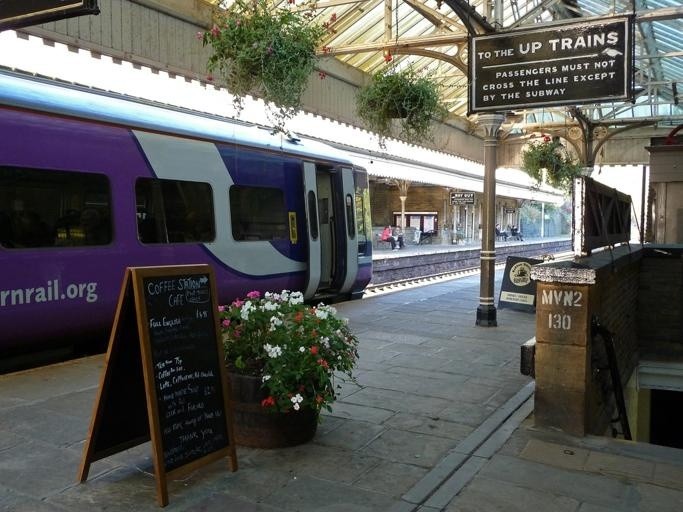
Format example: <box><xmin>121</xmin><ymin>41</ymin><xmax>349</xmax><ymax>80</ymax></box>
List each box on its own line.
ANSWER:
<box><xmin>375</xmin><ymin>233</ymin><xmax>398</xmax><ymax>250</ymax></box>
<box><xmin>495</xmin><ymin>230</ymin><xmax>521</xmax><ymax>242</ymax></box>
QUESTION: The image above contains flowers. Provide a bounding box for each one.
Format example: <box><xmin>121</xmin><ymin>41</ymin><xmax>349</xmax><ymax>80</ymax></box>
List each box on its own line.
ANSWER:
<box><xmin>518</xmin><ymin>134</ymin><xmax>580</xmax><ymax>191</ymax></box>
<box><xmin>214</xmin><ymin>284</ymin><xmax>363</xmax><ymax>416</ymax></box>
<box><xmin>352</xmin><ymin>53</ymin><xmax>446</xmax><ymax>149</ymax></box>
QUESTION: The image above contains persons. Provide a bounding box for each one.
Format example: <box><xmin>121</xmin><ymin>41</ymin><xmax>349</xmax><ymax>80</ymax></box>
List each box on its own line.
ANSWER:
<box><xmin>0</xmin><ymin>193</ymin><xmax>111</xmax><ymax>246</ymax></box>
<box><xmin>495</xmin><ymin>223</ymin><xmax>524</xmax><ymax>242</ymax></box>
<box><xmin>380</xmin><ymin>224</ymin><xmax>399</xmax><ymax>252</ymax></box>
<box><xmin>166</xmin><ymin>206</ymin><xmax>212</xmax><ymax>243</ymax></box>
<box><xmin>391</xmin><ymin>225</ymin><xmax>406</xmax><ymax>250</ymax></box>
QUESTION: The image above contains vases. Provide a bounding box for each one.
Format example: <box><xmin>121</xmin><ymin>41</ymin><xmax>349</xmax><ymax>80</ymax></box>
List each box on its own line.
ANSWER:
<box><xmin>227</xmin><ymin>371</ymin><xmax>320</xmax><ymax>450</ymax></box>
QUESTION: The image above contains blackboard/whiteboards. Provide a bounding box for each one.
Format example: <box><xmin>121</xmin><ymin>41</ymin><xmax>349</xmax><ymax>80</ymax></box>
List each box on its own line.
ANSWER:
<box><xmin>97</xmin><ymin>272</ymin><xmax>153</xmax><ymax>452</ymax></box>
<box><xmin>142</xmin><ymin>273</ymin><xmax>231</xmax><ymax>474</ymax></box>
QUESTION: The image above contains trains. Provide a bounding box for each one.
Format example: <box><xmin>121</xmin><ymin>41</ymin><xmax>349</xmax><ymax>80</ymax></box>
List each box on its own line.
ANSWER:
<box><xmin>0</xmin><ymin>70</ymin><xmax>372</xmax><ymax>352</ymax></box>
